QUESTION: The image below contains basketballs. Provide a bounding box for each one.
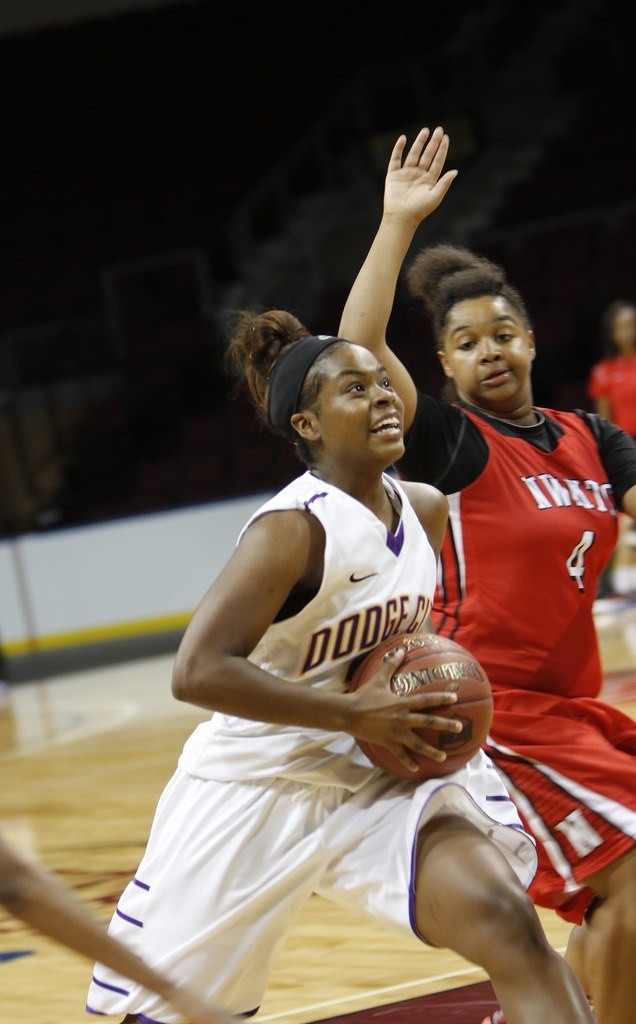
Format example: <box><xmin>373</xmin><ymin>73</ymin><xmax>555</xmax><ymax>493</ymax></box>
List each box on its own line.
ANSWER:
<box><xmin>348</xmin><ymin>632</ymin><xmax>495</xmax><ymax>780</ymax></box>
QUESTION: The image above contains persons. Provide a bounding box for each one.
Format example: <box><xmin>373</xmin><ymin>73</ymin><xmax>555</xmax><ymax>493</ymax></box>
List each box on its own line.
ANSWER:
<box><xmin>338</xmin><ymin>127</ymin><xmax>636</xmax><ymax>1024</ymax></box>
<box><xmin>84</xmin><ymin>312</ymin><xmax>592</xmax><ymax>1024</ymax></box>
<box><xmin>0</xmin><ymin>830</ymin><xmax>240</xmax><ymax>1024</ymax></box>
